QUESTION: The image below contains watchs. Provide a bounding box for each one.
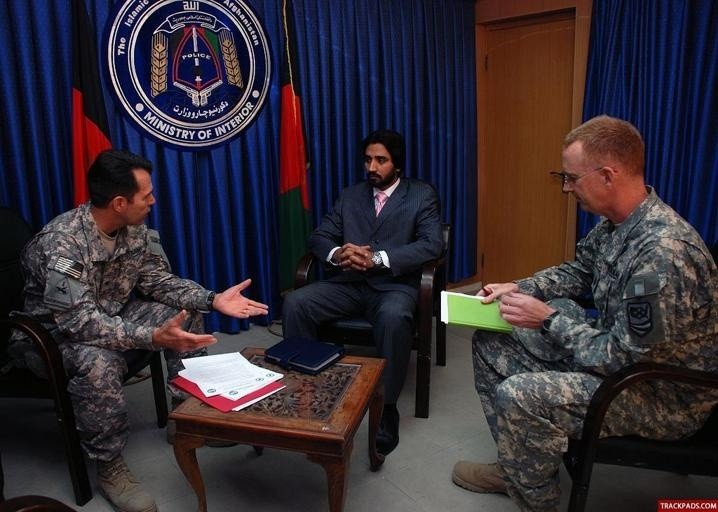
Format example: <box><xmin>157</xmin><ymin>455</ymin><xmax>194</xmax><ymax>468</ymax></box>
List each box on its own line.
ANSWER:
<box><xmin>372</xmin><ymin>251</ymin><xmax>383</xmax><ymax>266</ymax></box>
<box><xmin>540</xmin><ymin>310</ymin><xmax>560</xmax><ymax>336</ymax></box>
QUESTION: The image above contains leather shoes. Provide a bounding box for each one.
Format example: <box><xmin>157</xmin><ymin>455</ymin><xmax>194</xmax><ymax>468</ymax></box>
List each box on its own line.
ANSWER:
<box><xmin>377</xmin><ymin>407</ymin><xmax>402</xmax><ymax>455</ymax></box>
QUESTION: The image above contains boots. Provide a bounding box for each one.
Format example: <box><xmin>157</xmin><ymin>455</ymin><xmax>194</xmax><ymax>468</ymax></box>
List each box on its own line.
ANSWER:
<box><xmin>95</xmin><ymin>456</ymin><xmax>157</xmax><ymax>512</ymax></box>
<box><xmin>453</xmin><ymin>455</ymin><xmax>516</xmax><ymax>497</ymax></box>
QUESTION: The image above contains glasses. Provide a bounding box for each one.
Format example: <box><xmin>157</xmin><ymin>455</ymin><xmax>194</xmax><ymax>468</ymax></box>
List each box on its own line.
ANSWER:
<box><xmin>563</xmin><ymin>167</ymin><xmax>601</xmax><ymax>186</ymax></box>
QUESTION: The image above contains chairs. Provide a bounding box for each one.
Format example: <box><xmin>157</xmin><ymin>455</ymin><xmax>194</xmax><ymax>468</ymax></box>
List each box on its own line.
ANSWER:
<box><xmin>566</xmin><ymin>363</ymin><xmax>717</xmax><ymax>511</ymax></box>
<box><xmin>298</xmin><ymin>223</ymin><xmax>452</xmax><ymax>417</ymax></box>
<box><xmin>0</xmin><ymin>495</ymin><xmax>77</xmax><ymax>512</ymax></box>
<box><xmin>1</xmin><ymin>225</ymin><xmax>168</xmax><ymax>506</ymax></box>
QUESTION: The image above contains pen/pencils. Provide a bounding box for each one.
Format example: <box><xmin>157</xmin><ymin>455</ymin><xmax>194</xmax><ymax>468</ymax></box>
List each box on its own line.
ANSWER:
<box><xmin>482</xmin><ymin>288</ymin><xmax>489</xmax><ymax>295</ymax></box>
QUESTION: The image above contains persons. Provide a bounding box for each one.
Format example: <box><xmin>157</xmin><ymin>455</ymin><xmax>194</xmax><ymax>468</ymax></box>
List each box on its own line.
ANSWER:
<box><xmin>281</xmin><ymin>126</ymin><xmax>445</xmax><ymax>458</ymax></box>
<box><xmin>452</xmin><ymin>112</ymin><xmax>717</xmax><ymax>512</ymax></box>
<box><xmin>7</xmin><ymin>147</ymin><xmax>269</xmax><ymax>511</ymax></box>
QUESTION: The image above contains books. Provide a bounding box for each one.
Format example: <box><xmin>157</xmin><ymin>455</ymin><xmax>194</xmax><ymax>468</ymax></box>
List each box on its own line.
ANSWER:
<box><xmin>264</xmin><ymin>336</ymin><xmax>346</xmax><ymax>375</ymax></box>
<box><xmin>439</xmin><ymin>289</ymin><xmax>513</xmax><ymax>334</ymax></box>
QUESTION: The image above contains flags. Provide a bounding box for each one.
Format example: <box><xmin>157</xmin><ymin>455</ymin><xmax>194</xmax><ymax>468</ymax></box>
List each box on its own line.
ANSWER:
<box><xmin>69</xmin><ymin>0</ymin><xmax>113</xmax><ymax>208</ymax></box>
<box><xmin>279</xmin><ymin>1</ymin><xmax>317</xmax><ymax>292</ymax></box>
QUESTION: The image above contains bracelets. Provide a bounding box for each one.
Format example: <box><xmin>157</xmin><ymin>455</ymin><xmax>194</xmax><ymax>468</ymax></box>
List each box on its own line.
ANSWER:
<box><xmin>206</xmin><ymin>291</ymin><xmax>215</xmax><ymax>311</ymax></box>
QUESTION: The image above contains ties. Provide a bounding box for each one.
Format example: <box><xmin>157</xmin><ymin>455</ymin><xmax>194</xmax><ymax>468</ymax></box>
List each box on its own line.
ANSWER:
<box><xmin>374</xmin><ymin>192</ymin><xmax>388</xmax><ymax>218</ymax></box>
